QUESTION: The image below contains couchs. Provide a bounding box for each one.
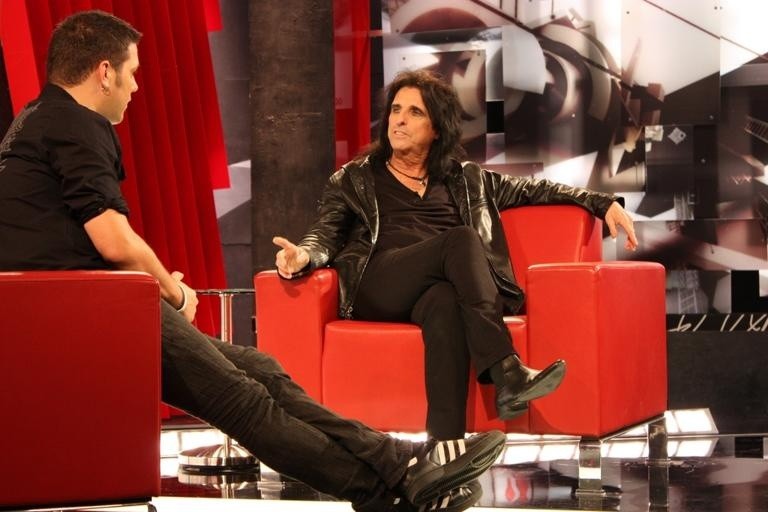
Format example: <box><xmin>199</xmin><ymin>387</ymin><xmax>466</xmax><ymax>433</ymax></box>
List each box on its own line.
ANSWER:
<box><xmin>255</xmin><ymin>204</ymin><xmax>665</xmax><ymax>497</ymax></box>
<box><xmin>1</xmin><ymin>272</ymin><xmax>161</xmax><ymax>507</ymax></box>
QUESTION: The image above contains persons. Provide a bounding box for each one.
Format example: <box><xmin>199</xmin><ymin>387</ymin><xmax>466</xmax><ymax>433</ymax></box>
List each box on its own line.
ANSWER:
<box><xmin>0</xmin><ymin>10</ymin><xmax>506</xmax><ymax>512</ymax></box>
<box><xmin>273</xmin><ymin>70</ymin><xmax>638</xmax><ymax>442</ymax></box>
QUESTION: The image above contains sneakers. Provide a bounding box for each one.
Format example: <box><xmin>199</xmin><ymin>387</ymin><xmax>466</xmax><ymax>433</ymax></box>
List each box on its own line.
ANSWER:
<box><xmin>358</xmin><ymin>430</ymin><xmax>506</xmax><ymax>512</ymax></box>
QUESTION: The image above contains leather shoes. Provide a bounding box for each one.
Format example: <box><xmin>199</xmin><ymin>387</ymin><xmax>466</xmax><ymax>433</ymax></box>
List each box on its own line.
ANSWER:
<box><xmin>495</xmin><ymin>359</ymin><xmax>567</xmax><ymax>420</ymax></box>
